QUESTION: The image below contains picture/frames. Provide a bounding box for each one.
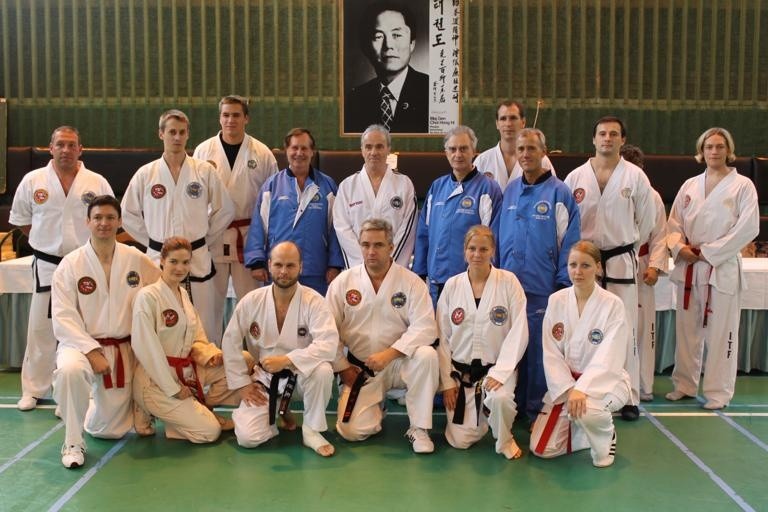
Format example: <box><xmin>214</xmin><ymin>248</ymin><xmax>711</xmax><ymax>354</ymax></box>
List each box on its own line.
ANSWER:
<box><xmin>338</xmin><ymin>3</ymin><xmax>463</xmax><ymax>139</ymax></box>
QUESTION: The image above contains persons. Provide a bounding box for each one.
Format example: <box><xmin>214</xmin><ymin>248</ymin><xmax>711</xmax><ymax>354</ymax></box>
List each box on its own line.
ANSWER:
<box><xmin>435</xmin><ymin>225</ymin><xmax>528</xmax><ymax>460</ymax></box>
<box><xmin>490</xmin><ymin>127</ymin><xmax>581</xmax><ymax>431</ymax></box>
<box><xmin>472</xmin><ymin>97</ymin><xmax>558</xmax><ymax>194</ymax></box>
<box><xmin>345</xmin><ymin>4</ymin><xmax>430</xmax><ymax>132</ymax></box>
<box><xmin>8</xmin><ymin>126</ymin><xmax>118</xmax><ymax>418</ymax></box>
<box><xmin>130</xmin><ymin>236</ymin><xmax>254</xmax><ymax>445</ymax></box>
<box><xmin>51</xmin><ymin>194</ymin><xmax>166</xmax><ymax>472</ymax></box>
<box><xmin>616</xmin><ymin>144</ymin><xmax>670</xmax><ymax>402</ymax></box>
<box><xmin>528</xmin><ymin>240</ymin><xmax>632</xmax><ymax>468</ymax></box>
<box><xmin>221</xmin><ymin>241</ymin><xmax>339</xmax><ymax>458</ymax></box>
<box><xmin>411</xmin><ymin>125</ymin><xmax>503</xmax><ymax>308</ymax></box>
<box><xmin>663</xmin><ymin>127</ymin><xmax>759</xmax><ymax>410</ymax></box>
<box><xmin>560</xmin><ymin>117</ymin><xmax>656</xmax><ymax>421</ymax></box>
<box><xmin>193</xmin><ymin>94</ymin><xmax>279</xmax><ymax>349</ymax></box>
<box><xmin>331</xmin><ymin>124</ymin><xmax>418</xmax><ymax>271</ymax></box>
<box><xmin>120</xmin><ymin>108</ymin><xmax>235</xmax><ymax>349</ymax></box>
<box><xmin>243</xmin><ymin>128</ymin><xmax>345</xmax><ymax>297</ymax></box>
<box><xmin>324</xmin><ymin>218</ymin><xmax>438</xmax><ymax>453</ymax></box>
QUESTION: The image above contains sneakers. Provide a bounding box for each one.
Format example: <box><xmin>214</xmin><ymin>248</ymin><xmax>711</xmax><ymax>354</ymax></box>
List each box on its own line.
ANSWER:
<box><xmin>213</xmin><ymin>411</ymin><xmax>233</xmax><ymax>430</ymax></box>
<box><xmin>17</xmin><ymin>394</ymin><xmax>37</xmax><ymax>411</ymax></box>
<box><xmin>621</xmin><ymin>405</ymin><xmax>639</xmax><ymax>420</ymax></box>
<box><xmin>592</xmin><ymin>431</ymin><xmax>617</xmax><ymax>467</ymax></box>
<box><xmin>703</xmin><ymin>400</ymin><xmax>727</xmax><ymax>409</ymax></box>
<box><xmin>640</xmin><ymin>389</ymin><xmax>653</xmax><ymax>401</ymax></box>
<box><xmin>666</xmin><ymin>390</ymin><xmax>686</xmax><ymax>401</ymax></box>
<box><xmin>133</xmin><ymin>400</ymin><xmax>156</xmax><ymax>436</ymax></box>
<box><xmin>406</xmin><ymin>427</ymin><xmax>434</xmax><ymax>453</ymax></box>
<box><xmin>60</xmin><ymin>441</ymin><xmax>85</xmax><ymax>468</ymax></box>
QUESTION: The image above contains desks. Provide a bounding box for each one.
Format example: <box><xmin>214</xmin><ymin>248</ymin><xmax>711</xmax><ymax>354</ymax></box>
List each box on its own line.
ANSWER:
<box><xmin>652</xmin><ymin>258</ymin><xmax>766</xmax><ymax>374</ymax></box>
<box><xmin>0</xmin><ymin>256</ymin><xmax>37</xmax><ymax>374</ymax></box>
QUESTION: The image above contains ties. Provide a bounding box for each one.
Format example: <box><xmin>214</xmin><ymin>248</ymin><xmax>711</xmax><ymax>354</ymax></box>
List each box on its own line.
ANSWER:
<box><xmin>379</xmin><ymin>89</ymin><xmax>393</xmax><ymax>131</ymax></box>
